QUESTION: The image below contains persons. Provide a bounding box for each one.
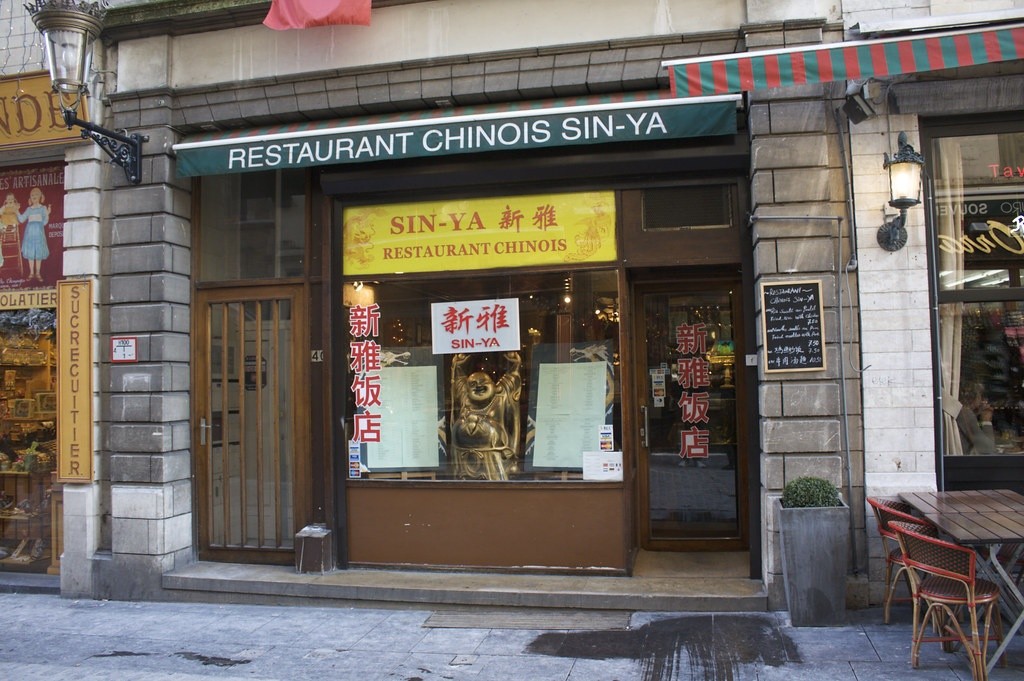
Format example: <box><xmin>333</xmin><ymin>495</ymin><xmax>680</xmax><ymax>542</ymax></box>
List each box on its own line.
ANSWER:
<box><xmin>449</xmin><ymin>351</ymin><xmax>522</xmax><ymax>481</ymax></box>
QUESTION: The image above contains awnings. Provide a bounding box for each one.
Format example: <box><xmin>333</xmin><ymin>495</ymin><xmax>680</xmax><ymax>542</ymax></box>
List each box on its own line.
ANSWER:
<box><xmin>660</xmin><ymin>23</ymin><xmax>1023</xmax><ymax>100</ymax></box>
<box><xmin>170</xmin><ymin>87</ymin><xmax>746</xmax><ymax>184</ymax></box>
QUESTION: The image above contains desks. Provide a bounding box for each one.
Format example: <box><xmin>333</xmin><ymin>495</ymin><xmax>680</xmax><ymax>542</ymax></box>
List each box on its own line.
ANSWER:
<box><xmin>897</xmin><ymin>488</ymin><xmax>1024</xmax><ymax>674</ymax></box>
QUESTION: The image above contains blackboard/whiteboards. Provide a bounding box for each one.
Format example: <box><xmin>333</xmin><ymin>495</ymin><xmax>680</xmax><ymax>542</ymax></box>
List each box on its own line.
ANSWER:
<box><xmin>760</xmin><ymin>280</ymin><xmax>827</xmax><ymax>374</ymax></box>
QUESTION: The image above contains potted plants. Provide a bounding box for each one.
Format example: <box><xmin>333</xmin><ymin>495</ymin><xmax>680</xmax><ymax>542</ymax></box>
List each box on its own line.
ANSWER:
<box><xmin>773</xmin><ymin>475</ymin><xmax>850</xmax><ymax>626</ymax></box>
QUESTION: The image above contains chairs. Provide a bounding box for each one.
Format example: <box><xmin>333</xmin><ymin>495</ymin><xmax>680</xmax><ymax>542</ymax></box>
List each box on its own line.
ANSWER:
<box><xmin>865</xmin><ymin>496</ymin><xmax>940</xmax><ymax>624</ymax></box>
<box><xmin>888</xmin><ymin>521</ymin><xmax>1008</xmax><ymax>681</ymax></box>
<box><xmin>983</xmin><ymin>544</ymin><xmax>1024</xmax><ymax>586</ymax></box>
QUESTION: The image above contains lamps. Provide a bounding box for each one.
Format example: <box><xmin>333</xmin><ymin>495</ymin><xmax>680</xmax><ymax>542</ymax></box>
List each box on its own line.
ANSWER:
<box><xmin>877</xmin><ymin>131</ymin><xmax>926</xmax><ymax>252</ymax></box>
<box><xmin>842</xmin><ymin>97</ymin><xmax>877</xmax><ymax>124</ymax></box>
<box><xmin>969</xmin><ymin>221</ymin><xmax>991</xmax><ymax>235</ymax></box>
<box><xmin>22</xmin><ymin>0</ymin><xmax>149</xmax><ymax>187</ymax></box>
<box><xmin>343</xmin><ymin>283</ymin><xmax>375</xmax><ymax>309</ymax></box>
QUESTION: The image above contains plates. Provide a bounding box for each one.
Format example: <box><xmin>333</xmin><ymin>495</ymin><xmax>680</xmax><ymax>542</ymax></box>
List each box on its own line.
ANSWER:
<box><xmin>1006</xmin><ymin>451</ymin><xmax>1024</xmax><ymax>455</ymax></box>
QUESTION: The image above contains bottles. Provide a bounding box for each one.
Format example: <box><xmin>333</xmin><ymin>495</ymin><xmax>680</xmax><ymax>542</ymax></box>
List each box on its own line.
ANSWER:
<box><xmin>992</xmin><ymin>406</ymin><xmax>1024</xmax><ymax>436</ymax></box>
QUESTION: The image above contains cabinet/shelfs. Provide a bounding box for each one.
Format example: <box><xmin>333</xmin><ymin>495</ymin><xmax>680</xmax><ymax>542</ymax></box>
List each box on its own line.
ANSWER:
<box><xmin>0</xmin><ymin>327</ymin><xmax>57</xmax><ymax>565</ymax></box>
